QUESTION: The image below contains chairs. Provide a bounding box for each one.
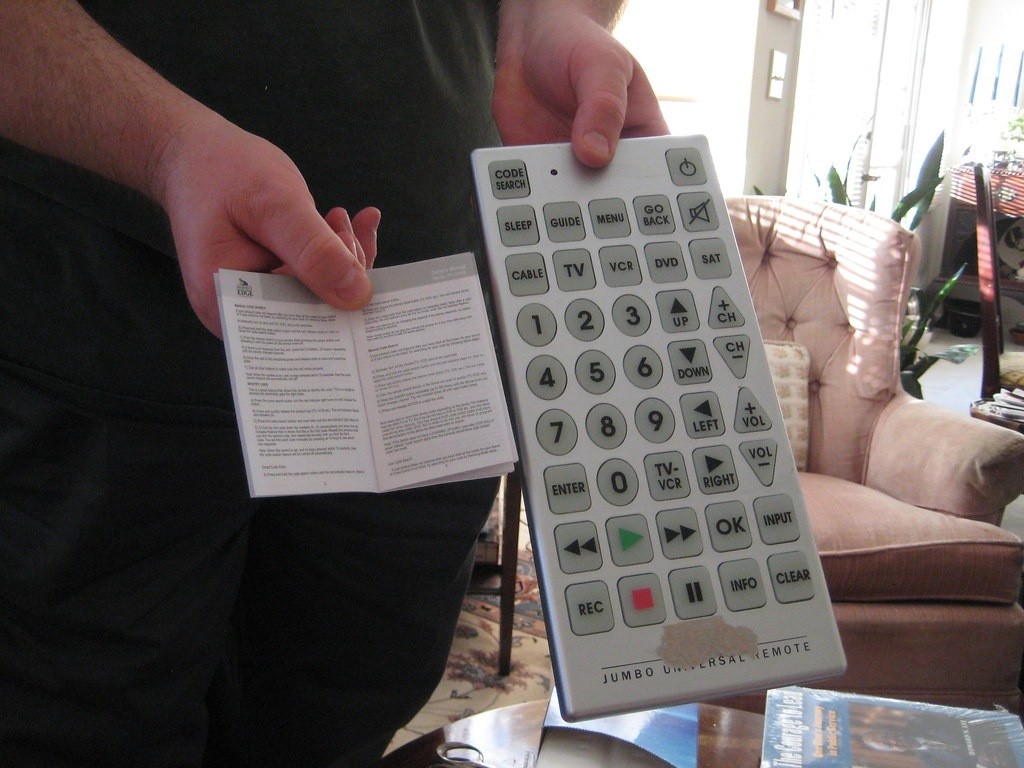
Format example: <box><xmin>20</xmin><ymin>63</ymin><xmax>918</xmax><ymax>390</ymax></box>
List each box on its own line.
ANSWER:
<box><xmin>973</xmin><ymin>164</ymin><xmax>1024</xmax><ymax>399</ymax></box>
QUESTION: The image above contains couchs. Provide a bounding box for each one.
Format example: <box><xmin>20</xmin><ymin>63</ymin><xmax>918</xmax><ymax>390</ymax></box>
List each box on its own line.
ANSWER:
<box><xmin>697</xmin><ymin>194</ymin><xmax>1024</xmax><ymax>722</ymax></box>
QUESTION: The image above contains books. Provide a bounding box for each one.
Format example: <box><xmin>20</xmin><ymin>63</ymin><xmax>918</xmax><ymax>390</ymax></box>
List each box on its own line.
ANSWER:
<box><xmin>211</xmin><ymin>250</ymin><xmax>519</xmax><ymax>499</ymax></box>
<box><xmin>760</xmin><ymin>686</ymin><xmax>1024</xmax><ymax>768</ymax></box>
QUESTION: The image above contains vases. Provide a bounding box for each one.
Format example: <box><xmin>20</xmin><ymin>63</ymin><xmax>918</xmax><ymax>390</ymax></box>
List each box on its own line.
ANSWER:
<box><xmin>945</xmin><ymin>297</ymin><xmax>984</xmax><ymax>338</ymax></box>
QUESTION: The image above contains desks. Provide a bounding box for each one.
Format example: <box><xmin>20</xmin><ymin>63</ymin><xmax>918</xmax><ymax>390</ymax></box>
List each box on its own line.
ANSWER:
<box><xmin>380</xmin><ymin>701</ymin><xmax>765</xmax><ymax>768</ymax></box>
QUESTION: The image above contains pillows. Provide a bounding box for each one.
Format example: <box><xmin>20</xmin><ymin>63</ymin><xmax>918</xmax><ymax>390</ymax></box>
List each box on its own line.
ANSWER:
<box><xmin>761</xmin><ymin>340</ymin><xmax>810</xmax><ymax>474</ymax></box>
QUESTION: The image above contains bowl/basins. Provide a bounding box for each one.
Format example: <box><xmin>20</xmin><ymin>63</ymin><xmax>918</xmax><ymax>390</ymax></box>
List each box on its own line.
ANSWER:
<box><xmin>944</xmin><ymin>303</ymin><xmax>982</xmax><ymax>338</ymax></box>
<box><xmin>1008</xmin><ymin>327</ymin><xmax>1024</xmax><ymax>344</ymax></box>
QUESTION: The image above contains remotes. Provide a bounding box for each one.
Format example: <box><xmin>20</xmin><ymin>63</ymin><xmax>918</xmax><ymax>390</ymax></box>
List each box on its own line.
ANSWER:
<box><xmin>471</xmin><ymin>134</ymin><xmax>848</xmax><ymax>725</ymax></box>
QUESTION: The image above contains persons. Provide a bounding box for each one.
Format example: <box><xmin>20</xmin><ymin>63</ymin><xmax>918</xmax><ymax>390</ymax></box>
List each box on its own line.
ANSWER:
<box><xmin>1</xmin><ymin>0</ymin><xmax>674</xmax><ymax>766</ymax></box>
